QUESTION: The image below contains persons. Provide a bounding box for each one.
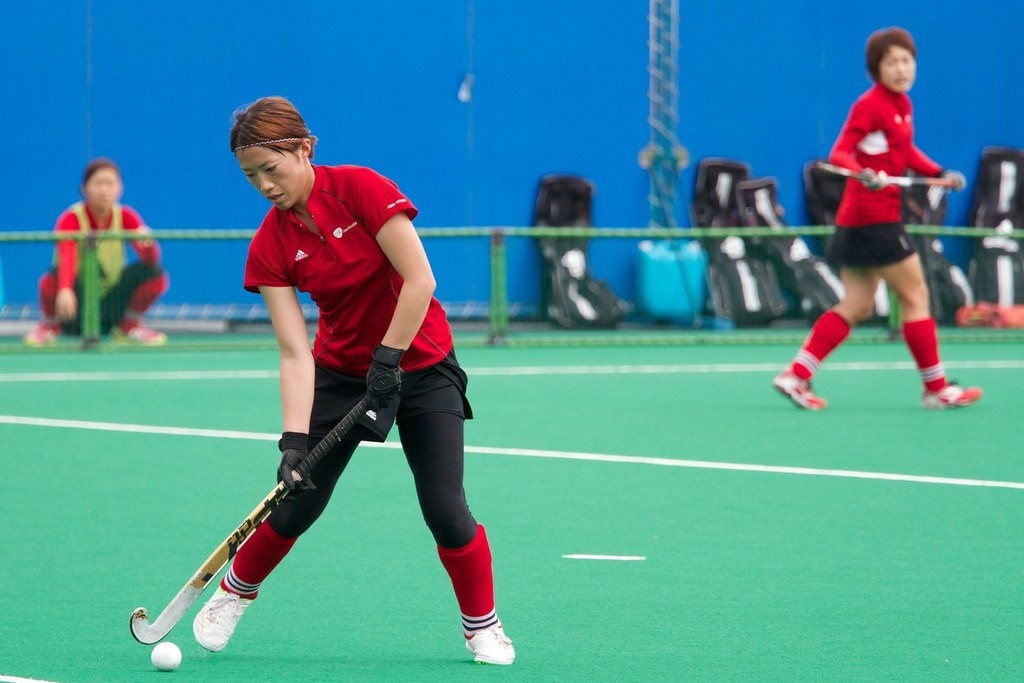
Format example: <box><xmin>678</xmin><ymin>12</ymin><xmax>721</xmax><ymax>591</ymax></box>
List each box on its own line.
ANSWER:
<box><xmin>192</xmin><ymin>97</ymin><xmax>517</xmax><ymax>666</ymax></box>
<box><xmin>25</xmin><ymin>160</ymin><xmax>166</xmax><ymax>352</ymax></box>
<box><xmin>773</xmin><ymin>29</ymin><xmax>984</xmax><ymax>411</ymax></box>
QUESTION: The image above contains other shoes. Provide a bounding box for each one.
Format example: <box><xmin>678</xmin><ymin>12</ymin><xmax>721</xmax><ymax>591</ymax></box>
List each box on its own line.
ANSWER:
<box><xmin>24</xmin><ymin>322</ymin><xmax>65</xmax><ymax>348</ymax></box>
<box><xmin>109</xmin><ymin>323</ymin><xmax>165</xmax><ymax>347</ymax></box>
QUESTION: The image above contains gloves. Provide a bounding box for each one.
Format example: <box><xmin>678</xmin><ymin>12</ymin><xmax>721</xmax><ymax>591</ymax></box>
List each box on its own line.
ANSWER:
<box><xmin>366</xmin><ymin>343</ymin><xmax>403</xmax><ymax>412</ymax></box>
<box><xmin>940</xmin><ymin>170</ymin><xmax>967</xmax><ymax>192</ymax></box>
<box><xmin>862</xmin><ymin>168</ymin><xmax>887</xmax><ymax>190</ymax></box>
<box><xmin>278</xmin><ymin>431</ymin><xmax>317</xmax><ymax>504</ymax></box>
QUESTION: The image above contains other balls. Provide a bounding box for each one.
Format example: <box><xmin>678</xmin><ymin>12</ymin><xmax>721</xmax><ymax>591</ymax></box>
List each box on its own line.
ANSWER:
<box><xmin>150</xmin><ymin>641</ymin><xmax>182</xmax><ymax>671</ymax></box>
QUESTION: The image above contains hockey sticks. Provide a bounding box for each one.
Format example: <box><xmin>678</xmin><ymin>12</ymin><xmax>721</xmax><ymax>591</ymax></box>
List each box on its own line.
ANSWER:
<box><xmin>129</xmin><ymin>394</ymin><xmax>372</xmax><ymax>648</ymax></box>
<box><xmin>814</xmin><ymin>163</ymin><xmax>958</xmax><ymax>190</ymax></box>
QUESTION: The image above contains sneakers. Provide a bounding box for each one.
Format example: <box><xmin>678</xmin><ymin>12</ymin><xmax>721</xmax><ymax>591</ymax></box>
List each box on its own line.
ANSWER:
<box><xmin>923</xmin><ymin>383</ymin><xmax>982</xmax><ymax>409</ymax></box>
<box><xmin>465</xmin><ymin>628</ymin><xmax>515</xmax><ymax>665</ymax></box>
<box><xmin>193</xmin><ymin>584</ymin><xmax>253</xmax><ymax>651</ymax></box>
<box><xmin>774</xmin><ymin>366</ymin><xmax>826</xmax><ymax>411</ymax></box>
